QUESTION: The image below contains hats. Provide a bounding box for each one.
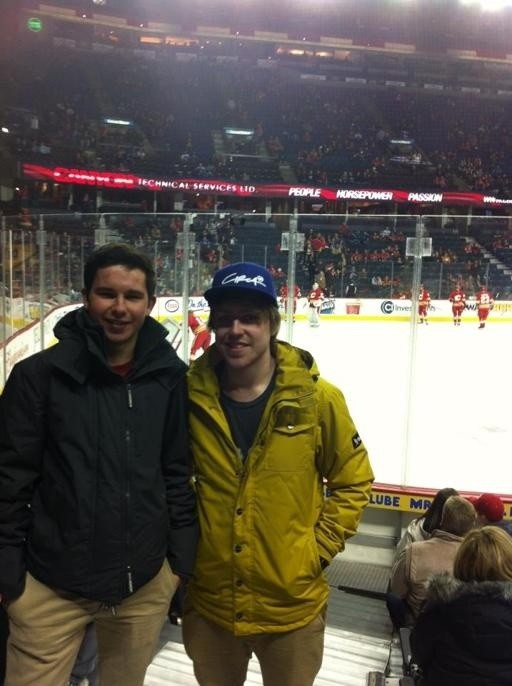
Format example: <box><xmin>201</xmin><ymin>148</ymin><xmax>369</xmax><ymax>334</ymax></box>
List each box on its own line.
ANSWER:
<box><xmin>204</xmin><ymin>262</ymin><xmax>277</xmax><ymax>301</ymax></box>
<box><xmin>469</xmin><ymin>493</ymin><xmax>504</xmax><ymax>522</ymax></box>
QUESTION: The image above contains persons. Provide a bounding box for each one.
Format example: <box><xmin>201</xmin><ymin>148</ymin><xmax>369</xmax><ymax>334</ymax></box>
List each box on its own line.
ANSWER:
<box><xmin>306</xmin><ymin>280</ymin><xmax>326</xmax><ymax>328</ymax></box>
<box><xmin>1</xmin><ymin>0</ymin><xmax>512</xmax><ymax>300</ymax></box>
<box><xmin>408</xmin><ymin>525</ymin><xmax>511</xmax><ymax>685</ymax></box>
<box><xmin>385</xmin><ymin>496</ymin><xmax>477</xmax><ymax>632</ymax></box>
<box><xmin>474</xmin><ymin>285</ymin><xmax>494</xmax><ymax>328</ymax></box>
<box><xmin>180</xmin><ymin>260</ymin><xmax>375</xmax><ymax>685</ymax></box>
<box><xmin>396</xmin><ymin>488</ymin><xmax>461</xmax><ymax>553</ymax></box>
<box><xmin>417</xmin><ymin>283</ymin><xmax>432</xmax><ymax>325</ymax></box>
<box><xmin>0</xmin><ymin>240</ymin><xmax>202</xmax><ymax>684</ymax></box>
<box><xmin>468</xmin><ymin>490</ymin><xmax>512</xmax><ymax>536</ymax></box>
<box><xmin>447</xmin><ymin>282</ymin><xmax>467</xmax><ymax>327</ymax></box>
<box><xmin>278</xmin><ymin>277</ymin><xmax>302</xmax><ymax>323</ymax></box>
